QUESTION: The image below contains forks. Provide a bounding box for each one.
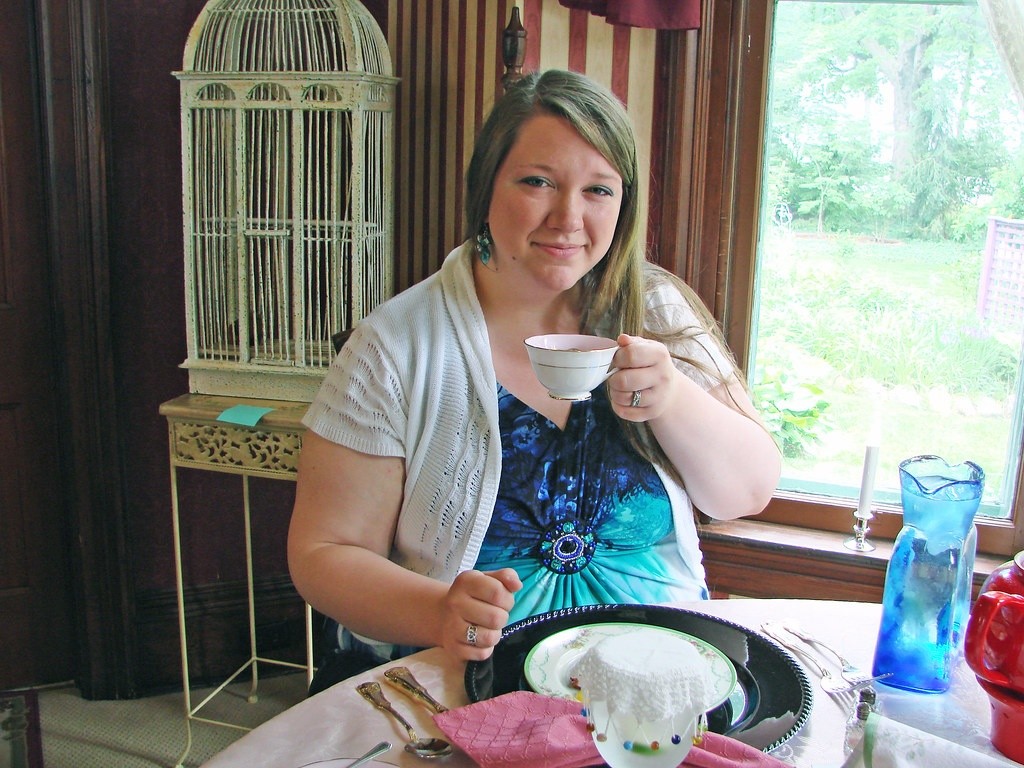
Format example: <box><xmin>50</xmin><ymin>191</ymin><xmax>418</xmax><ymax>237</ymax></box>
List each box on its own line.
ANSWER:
<box><xmin>758</xmin><ymin>620</ymin><xmax>895</xmax><ymax>693</ymax></box>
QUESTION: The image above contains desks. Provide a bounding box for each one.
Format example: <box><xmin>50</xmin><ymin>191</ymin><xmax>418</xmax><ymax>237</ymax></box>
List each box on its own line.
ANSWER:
<box><xmin>159</xmin><ymin>394</ymin><xmax>363</xmax><ymax>768</ymax></box>
<box><xmin>200</xmin><ymin>598</ymin><xmax>1024</xmax><ymax>768</ymax></box>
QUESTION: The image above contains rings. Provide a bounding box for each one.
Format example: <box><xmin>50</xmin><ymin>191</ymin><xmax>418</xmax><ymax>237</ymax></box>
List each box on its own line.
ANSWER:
<box><xmin>631</xmin><ymin>390</ymin><xmax>642</xmax><ymax>407</ymax></box>
<box><xmin>467</xmin><ymin>624</ymin><xmax>477</xmax><ymax>644</ymax></box>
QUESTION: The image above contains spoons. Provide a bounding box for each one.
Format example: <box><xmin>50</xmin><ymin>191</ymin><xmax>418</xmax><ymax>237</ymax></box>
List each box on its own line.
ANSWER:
<box><xmin>355</xmin><ymin>681</ymin><xmax>453</xmax><ymax>761</ymax></box>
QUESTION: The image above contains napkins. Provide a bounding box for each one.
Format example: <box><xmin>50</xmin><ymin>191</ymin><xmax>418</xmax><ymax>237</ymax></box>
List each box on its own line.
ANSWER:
<box><xmin>843</xmin><ymin>650</ymin><xmax>1024</xmax><ymax>768</ymax></box>
<box><xmin>433</xmin><ymin>691</ymin><xmax>797</xmax><ymax>768</ymax></box>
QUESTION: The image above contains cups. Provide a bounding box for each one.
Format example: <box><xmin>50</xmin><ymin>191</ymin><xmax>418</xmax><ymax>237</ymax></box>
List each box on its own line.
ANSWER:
<box><xmin>523</xmin><ymin>333</ymin><xmax>623</xmax><ymax>402</ymax></box>
<box><xmin>584</xmin><ymin>631</ymin><xmax>705</xmax><ymax>768</ymax></box>
<box><xmin>964</xmin><ymin>549</ymin><xmax>1024</xmax><ymax>693</ymax></box>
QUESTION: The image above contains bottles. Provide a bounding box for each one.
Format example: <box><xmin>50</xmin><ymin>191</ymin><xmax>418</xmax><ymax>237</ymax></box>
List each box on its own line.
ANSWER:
<box><xmin>873</xmin><ymin>454</ymin><xmax>986</xmax><ymax>694</ymax></box>
<box><xmin>844</xmin><ymin>689</ymin><xmax>875</xmax><ymax>755</ymax></box>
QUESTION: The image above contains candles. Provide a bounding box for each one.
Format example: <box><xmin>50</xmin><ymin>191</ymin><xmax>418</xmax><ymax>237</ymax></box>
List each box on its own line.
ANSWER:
<box><xmin>857</xmin><ymin>442</ymin><xmax>883</xmax><ymax>516</ymax></box>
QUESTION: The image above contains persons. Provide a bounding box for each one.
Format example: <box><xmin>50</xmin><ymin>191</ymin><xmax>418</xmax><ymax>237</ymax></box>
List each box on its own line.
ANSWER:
<box><xmin>286</xmin><ymin>71</ymin><xmax>780</xmax><ymax>660</ymax></box>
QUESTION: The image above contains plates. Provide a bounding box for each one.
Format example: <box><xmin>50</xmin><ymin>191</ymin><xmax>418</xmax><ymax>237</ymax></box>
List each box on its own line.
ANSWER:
<box><xmin>705</xmin><ymin>652</ymin><xmax>761</xmax><ymax>737</ymax></box>
<box><xmin>296</xmin><ymin>758</ymin><xmax>400</xmax><ymax>768</ymax></box>
<box><xmin>524</xmin><ymin>621</ymin><xmax>737</xmax><ymax>718</ymax></box>
